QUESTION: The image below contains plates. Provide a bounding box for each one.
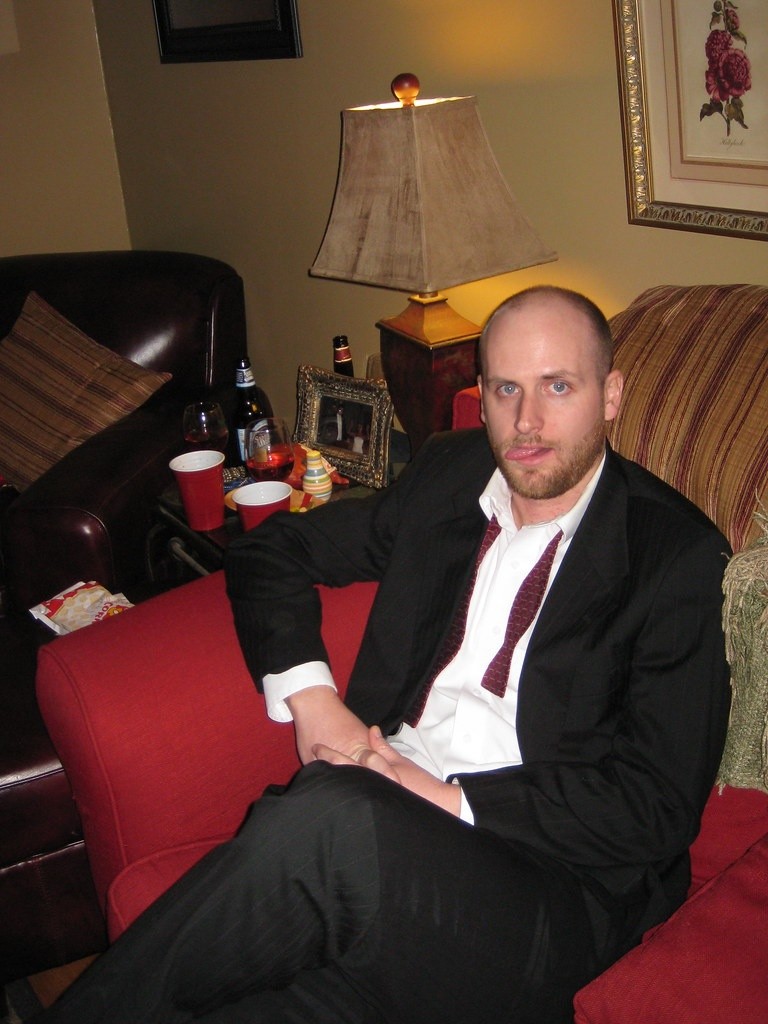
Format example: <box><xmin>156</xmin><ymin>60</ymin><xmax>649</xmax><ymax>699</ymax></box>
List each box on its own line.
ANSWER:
<box><xmin>224</xmin><ymin>485</ymin><xmax>323</xmax><ymax>513</ymax></box>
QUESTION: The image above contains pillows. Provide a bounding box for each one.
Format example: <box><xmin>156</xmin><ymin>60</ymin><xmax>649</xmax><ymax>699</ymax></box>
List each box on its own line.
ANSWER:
<box><xmin>606</xmin><ymin>284</ymin><xmax>768</xmax><ymax>553</ymax></box>
<box><xmin>0</xmin><ymin>290</ymin><xmax>172</xmax><ymax>495</ymax></box>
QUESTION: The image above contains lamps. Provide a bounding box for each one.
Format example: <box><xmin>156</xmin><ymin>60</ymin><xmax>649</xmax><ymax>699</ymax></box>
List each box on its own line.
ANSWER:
<box><xmin>307</xmin><ymin>73</ymin><xmax>558</xmax><ymax>458</ymax></box>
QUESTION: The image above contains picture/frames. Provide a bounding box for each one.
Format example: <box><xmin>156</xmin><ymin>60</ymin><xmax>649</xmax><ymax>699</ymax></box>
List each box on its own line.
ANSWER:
<box><xmin>611</xmin><ymin>0</ymin><xmax>768</xmax><ymax>241</ymax></box>
<box><xmin>293</xmin><ymin>365</ymin><xmax>393</xmax><ymax>490</ymax></box>
<box><xmin>151</xmin><ymin>0</ymin><xmax>303</xmax><ymax>65</ymax></box>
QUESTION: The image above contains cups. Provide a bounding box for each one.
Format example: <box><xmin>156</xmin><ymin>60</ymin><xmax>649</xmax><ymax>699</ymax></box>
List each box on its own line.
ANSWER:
<box><xmin>182</xmin><ymin>402</ymin><xmax>229</xmax><ymax>453</ymax></box>
<box><xmin>244</xmin><ymin>417</ymin><xmax>295</xmax><ymax>482</ymax></box>
<box><xmin>231</xmin><ymin>480</ymin><xmax>293</xmax><ymax>531</ymax></box>
<box><xmin>169</xmin><ymin>450</ymin><xmax>226</xmax><ymax>531</ymax></box>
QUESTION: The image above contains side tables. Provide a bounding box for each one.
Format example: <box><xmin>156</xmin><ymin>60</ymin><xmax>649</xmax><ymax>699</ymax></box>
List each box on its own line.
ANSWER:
<box><xmin>140</xmin><ymin>475</ymin><xmax>378</xmax><ymax>601</ymax></box>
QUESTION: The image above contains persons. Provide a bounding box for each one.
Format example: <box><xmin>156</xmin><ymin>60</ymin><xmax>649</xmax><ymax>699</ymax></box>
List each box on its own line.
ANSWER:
<box><xmin>36</xmin><ymin>287</ymin><xmax>735</xmax><ymax>1024</ymax></box>
<box><xmin>331</xmin><ymin>406</ymin><xmax>347</xmax><ymax>445</ymax></box>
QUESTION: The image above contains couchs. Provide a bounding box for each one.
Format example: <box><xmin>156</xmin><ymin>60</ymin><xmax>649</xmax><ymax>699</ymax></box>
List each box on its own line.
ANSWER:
<box><xmin>36</xmin><ymin>386</ymin><xmax>768</xmax><ymax>1024</ymax></box>
<box><xmin>0</xmin><ymin>247</ymin><xmax>247</xmax><ymax>967</ymax></box>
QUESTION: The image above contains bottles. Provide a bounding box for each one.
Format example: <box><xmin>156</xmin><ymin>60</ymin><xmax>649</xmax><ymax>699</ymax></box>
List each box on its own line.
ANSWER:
<box><xmin>332</xmin><ymin>335</ymin><xmax>354</xmax><ymax>378</ymax></box>
<box><xmin>302</xmin><ymin>451</ymin><xmax>332</xmax><ymax>503</ymax></box>
<box><xmin>224</xmin><ymin>356</ymin><xmax>273</xmax><ymax>470</ymax></box>
